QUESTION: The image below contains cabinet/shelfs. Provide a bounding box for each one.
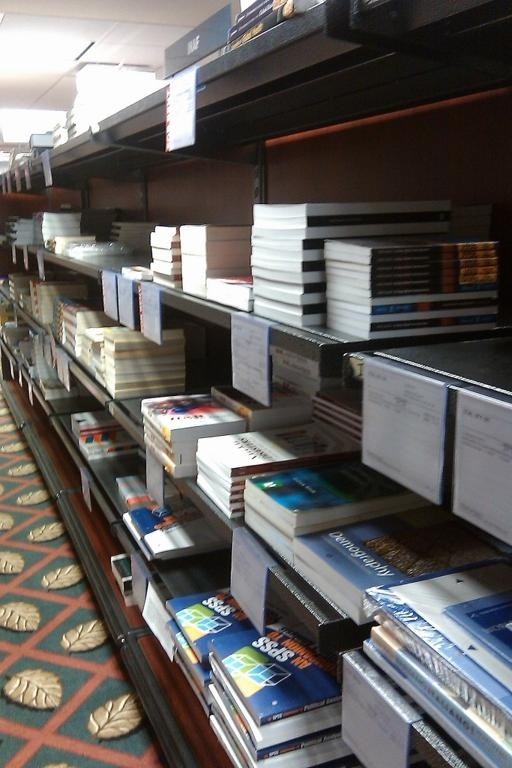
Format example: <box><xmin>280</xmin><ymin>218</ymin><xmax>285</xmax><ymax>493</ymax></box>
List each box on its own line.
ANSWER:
<box><xmin>0</xmin><ymin>2</ymin><xmax>512</xmax><ymax>768</ymax></box>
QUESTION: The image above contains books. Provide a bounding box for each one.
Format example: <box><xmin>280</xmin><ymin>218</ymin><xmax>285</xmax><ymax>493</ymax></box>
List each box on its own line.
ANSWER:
<box><xmin>206</xmin><ymin>276</ymin><xmax>253</xmax><ymax>311</ymax></box>
<box><xmin>179</xmin><ymin>225</ymin><xmax>251</xmax><ymax>300</ymax></box>
<box><xmin>109</xmin><ymin>222</ymin><xmax>160</xmax><ymax>244</ymax></box>
<box><xmin>150</xmin><ymin>223</ymin><xmax>183</xmax><ymax>288</ymax></box>
<box><xmin>226</xmin><ymin>1</ymin><xmax>321</xmax><ymax>53</ymax></box>
<box><xmin>0</xmin><ymin>272</ymin><xmax>123</xmax><ymax>400</ymax></box>
<box><xmin>111</xmin><ymin>552</ymin><xmax>135</xmax><ymax>608</ymax></box>
<box><xmin>252</xmin><ymin>201</ymin><xmax>496</xmax><ymax>327</ymax></box>
<box><xmin>72</xmin><ymin>412</ymin><xmax>139</xmax><ymax>460</ymax></box>
<box><xmin>62</xmin><ymin>241</ymin><xmax>125</xmax><ymax>258</ymax></box>
<box><xmin>117</xmin><ymin>476</ymin><xmax>233</xmax><ymax>561</ymax></box>
<box><xmin>364</xmin><ymin>557</ymin><xmax>511</xmax><ymax>766</ymax></box>
<box><xmin>52</xmin><ymin>109</ymin><xmax>79</xmax><ymax>148</ymax></box>
<box><xmin>206</xmin><ymin>617</ymin><xmax>354</xmax><ymax>768</ymax></box>
<box><xmin>29</xmin><ymin>133</ymin><xmax>54</xmax><ymax>156</ymax></box>
<box><xmin>165</xmin><ymin>588</ymin><xmax>283</xmax><ymax>716</ymax></box>
<box><xmin>48</xmin><ymin>235</ymin><xmax>95</xmax><ymax>255</ymax></box>
<box><xmin>323</xmin><ymin>232</ymin><xmax>498</xmax><ymax>337</ymax></box>
<box><xmin>120</xmin><ymin>265</ymin><xmax>152</xmax><ymax>280</ymax></box>
<box><xmin>293</xmin><ymin>505</ymin><xmax>502</xmax><ymax>625</ymax></box>
<box><xmin>243</xmin><ymin>461</ymin><xmax>432</xmax><ymax>563</ymax></box>
<box><xmin>142</xmin><ymin>385</ymin><xmax>314</xmax><ymax>480</ymax></box>
<box><xmin>103</xmin><ymin>329</ymin><xmax>186</xmax><ymax>400</ymax></box>
<box><xmin>196</xmin><ymin>421</ymin><xmax>359</xmax><ymax>520</ymax></box>
<box><xmin>316</xmin><ymin>388</ymin><xmax>362</xmax><ymax>440</ymax></box>
<box><xmin>31</xmin><ymin>211</ymin><xmax>81</xmax><ymax>249</ymax></box>
<box><xmin>6</xmin><ymin>218</ymin><xmax>33</xmax><ymax>246</ymax></box>
